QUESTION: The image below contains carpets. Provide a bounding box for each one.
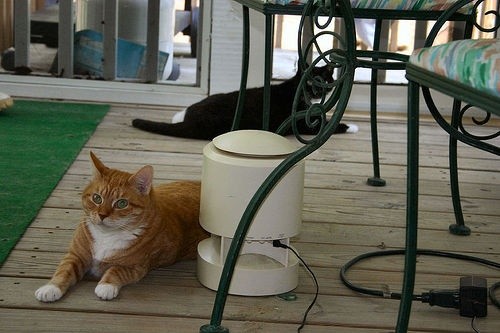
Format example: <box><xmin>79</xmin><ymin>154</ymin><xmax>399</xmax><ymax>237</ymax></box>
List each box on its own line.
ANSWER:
<box><xmin>0</xmin><ymin>98</ymin><xmax>113</xmax><ymax>268</ymax></box>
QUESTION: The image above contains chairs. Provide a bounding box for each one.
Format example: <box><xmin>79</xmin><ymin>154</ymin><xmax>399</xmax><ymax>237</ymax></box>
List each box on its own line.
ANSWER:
<box><xmin>395</xmin><ymin>7</ymin><xmax>498</xmax><ymax>333</ymax></box>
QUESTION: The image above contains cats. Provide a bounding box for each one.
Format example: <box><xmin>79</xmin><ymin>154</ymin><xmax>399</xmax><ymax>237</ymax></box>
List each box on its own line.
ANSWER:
<box><xmin>131</xmin><ymin>58</ymin><xmax>359</xmax><ymax>141</ymax></box>
<box><xmin>33</xmin><ymin>147</ymin><xmax>209</xmax><ymax>303</ymax></box>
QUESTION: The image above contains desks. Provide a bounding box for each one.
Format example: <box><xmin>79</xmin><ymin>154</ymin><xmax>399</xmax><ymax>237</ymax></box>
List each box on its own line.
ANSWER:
<box><xmin>197</xmin><ymin>0</ymin><xmax>485</xmax><ymax>333</ymax></box>
